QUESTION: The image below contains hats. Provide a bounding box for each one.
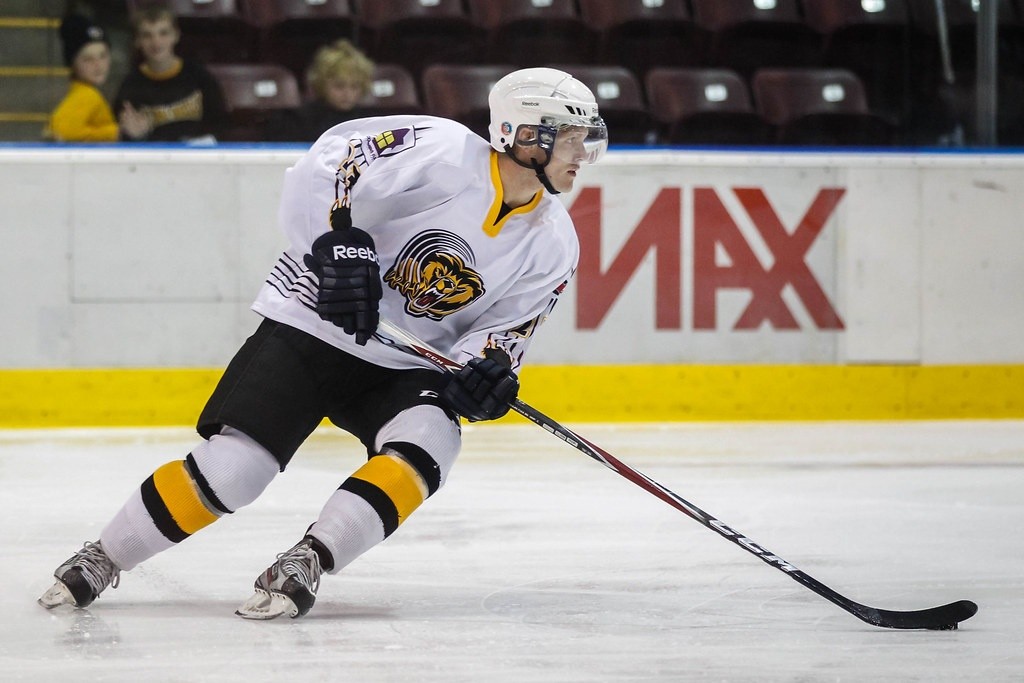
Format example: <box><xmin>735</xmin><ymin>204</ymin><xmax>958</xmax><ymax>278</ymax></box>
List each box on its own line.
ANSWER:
<box><xmin>55</xmin><ymin>12</ymin><xmax>113</xmax><ymax>68</ymax></box>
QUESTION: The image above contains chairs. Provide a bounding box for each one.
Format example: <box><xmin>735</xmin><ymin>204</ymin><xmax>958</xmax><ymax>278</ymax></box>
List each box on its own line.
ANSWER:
<box><xmin>126</xmin><ymin>0</ymin><xmax>921</xmax><ymax>153</ymax></box>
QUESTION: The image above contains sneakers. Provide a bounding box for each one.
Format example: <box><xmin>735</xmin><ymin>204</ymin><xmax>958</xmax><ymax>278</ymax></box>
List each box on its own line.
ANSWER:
<box><xmin>37</xmin><ymin>538</ymin><xmax>122</xmax><ymax>610</ymax></box>
<box><xmin>234</xmin><ymin>538</ymin><xmax>333</xmax><ymax>620</ymax></box>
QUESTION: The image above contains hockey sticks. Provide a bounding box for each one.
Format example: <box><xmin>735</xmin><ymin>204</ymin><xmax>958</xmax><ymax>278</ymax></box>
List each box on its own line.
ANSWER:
<box><xmin>375</xmin><ymin>317</ymin><xmax>979</xmax><ymax>630</ymax></box>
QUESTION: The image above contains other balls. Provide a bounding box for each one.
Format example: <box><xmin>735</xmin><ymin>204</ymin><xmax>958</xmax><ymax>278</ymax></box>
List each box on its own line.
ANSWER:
<box><xmin>927</xmin><ymin>620</ymin><xmax>960</xmax><ymax>631</ymax></box>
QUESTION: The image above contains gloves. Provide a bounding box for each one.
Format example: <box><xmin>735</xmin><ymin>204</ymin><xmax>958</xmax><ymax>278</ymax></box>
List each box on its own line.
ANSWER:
<box><xmin>442</xmin><ymin>357</ymin><xmax>520</xmax><ymax>422</ymax></box>
<box><xmin>303</xmin><ymin>227</ymin><xmax>384</xmax><ymax>346</ymax></box>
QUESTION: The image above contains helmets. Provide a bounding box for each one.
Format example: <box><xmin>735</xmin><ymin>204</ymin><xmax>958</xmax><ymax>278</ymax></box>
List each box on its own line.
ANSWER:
<box><xmin>488</xmin><ymin>66</ymin><xmax>599</xmax><ymax>154</ymax></box>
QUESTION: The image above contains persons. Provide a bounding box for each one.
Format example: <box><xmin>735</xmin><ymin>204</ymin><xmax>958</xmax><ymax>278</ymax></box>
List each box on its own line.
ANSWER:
<box><xmin>43</xmin><ymin>10</ymin><xmax>121</xmax><ymax>141</ymax></box>
<box><xmin>112</xmin><ymin>4</ymin><xmax>226</xmax><ymax>146</ymax></box>
<box><xmin>37</xmin><ymin>67</ymin><xmax>608</xmax><ymax>620</ymax></box>
<box><xmin>253</xmin><ymin>39</ymin><xmax>386</xmax><ymax>139</ymax></box>
